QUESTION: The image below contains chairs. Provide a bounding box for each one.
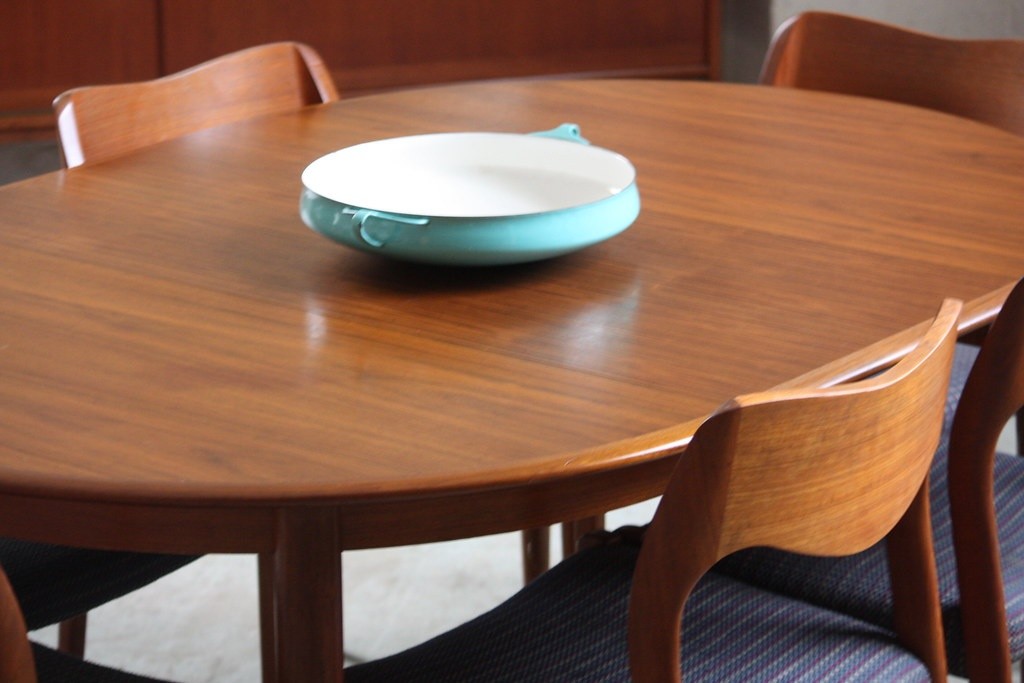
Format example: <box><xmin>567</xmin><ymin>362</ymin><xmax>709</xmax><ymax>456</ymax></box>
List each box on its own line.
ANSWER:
<box><xmin>756</xmin><ymin>8</ymin><xmax>1024</xmax><ymax>139</ymax></box>
<box><xmin>346</xmin><ymin>299</ymin><xmax>964</xmax><ymax>683</ymax></box>
<box><xmin>51</xmin><ymin>41</ymin><xmax>339</xmax><ymax>168</ymax></box>
<box><xmin>717</xmin><ymin>278</ymin><xmax>1024</xmax><ymax>683</ymax></box>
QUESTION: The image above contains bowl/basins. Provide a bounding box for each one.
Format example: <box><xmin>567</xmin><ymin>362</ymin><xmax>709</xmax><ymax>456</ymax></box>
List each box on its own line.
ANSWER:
<box><xmin>301</xmin><ymin>118</ymin><xmax>643</xmax><ymax>266</ymax></box>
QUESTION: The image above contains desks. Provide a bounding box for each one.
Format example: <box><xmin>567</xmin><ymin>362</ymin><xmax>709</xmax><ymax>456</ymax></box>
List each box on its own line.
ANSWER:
<box><xmin>0</xmin><ymin>78</ymin><xmax>1023</xmax><ymax>683</ymax></box>
